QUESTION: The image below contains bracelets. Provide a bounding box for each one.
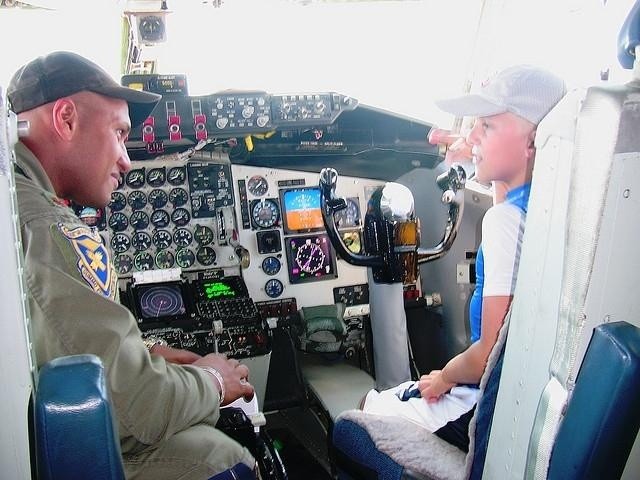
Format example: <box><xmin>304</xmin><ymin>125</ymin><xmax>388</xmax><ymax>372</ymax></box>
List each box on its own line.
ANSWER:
<box><xmin>198</xmin><ymin>365</ymin><xmax>226</xmax><ymax>404</ymax></box>
<box><xmin>143</xmin><ymin>334</ymin><xmax>163</xmax><ymax>350</ymax></box>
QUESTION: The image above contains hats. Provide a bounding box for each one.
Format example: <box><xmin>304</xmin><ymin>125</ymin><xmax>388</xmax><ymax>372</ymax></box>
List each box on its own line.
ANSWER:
<box><xmin>435</xmin><ymin>65</ymin><xmax>569</xmax><ymax>124</ymax></box>
<box><xmin>6</xmin><ymin>52</ymin><xmax>162</xmax><ymax>130</ymax></box>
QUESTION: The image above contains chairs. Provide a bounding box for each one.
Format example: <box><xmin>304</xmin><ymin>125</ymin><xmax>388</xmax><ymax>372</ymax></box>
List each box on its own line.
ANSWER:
<box><xmin>337</xmin><ymin>74</ymin><xmax>640</xmax><ymax>480</ymax></box>
<box><xmin>0</xmin><ymin>85</ymin><xmax>123</xmax><ymax>479</ymax></box>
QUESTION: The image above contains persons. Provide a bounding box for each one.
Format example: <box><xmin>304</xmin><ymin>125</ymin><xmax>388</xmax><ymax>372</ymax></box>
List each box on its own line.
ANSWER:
<box><xmin>6</xmin><ymin>49</ymin><xmax>262</xmax><ymax>480</ymax></box>
<box><xmin>359</xmin><ymin>67</ymin><xmax>568</xmax><ymax>448</ymax></box>
